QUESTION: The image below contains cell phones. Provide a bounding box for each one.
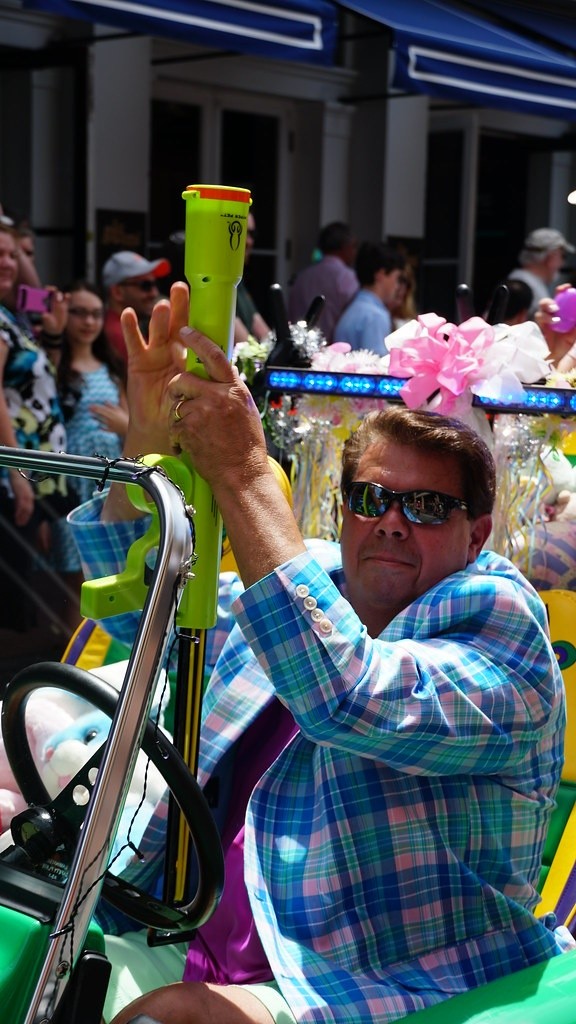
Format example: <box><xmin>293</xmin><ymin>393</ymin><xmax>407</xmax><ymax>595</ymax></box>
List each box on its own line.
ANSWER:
<box><xmin>16</xmin><ymin>284</ymin><xmax>55</xmax><ymax>314</ymax></box>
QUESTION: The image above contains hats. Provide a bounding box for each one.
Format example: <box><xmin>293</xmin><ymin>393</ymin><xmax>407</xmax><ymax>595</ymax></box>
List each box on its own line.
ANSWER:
<box><xmin>525</xmin><ymin>228</ymin><xmax>575</xmax><ymax>255</ymax></box>
<box><xmin>102</xmin><ymin>251</ymin><xmax>171</xmax><ymax>287</ymax></box>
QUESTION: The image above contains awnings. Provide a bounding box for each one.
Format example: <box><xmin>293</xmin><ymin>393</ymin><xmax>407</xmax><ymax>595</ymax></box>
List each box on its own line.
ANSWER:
<box><xmin>21</xmin><ymin>0</ymin><xmax>339</xmax><ymax>68</ymax></box>
<box><xmin>342</xmin><ymin>0</ymin><xmax>576</xmax><ymax>121</ymax></box>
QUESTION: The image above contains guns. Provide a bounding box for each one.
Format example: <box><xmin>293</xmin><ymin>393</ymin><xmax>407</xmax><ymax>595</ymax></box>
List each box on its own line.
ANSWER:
<box><xmin>80</xmin><ymin>180</ymin><xmax>253</xmax><ymax>948</ymax></box>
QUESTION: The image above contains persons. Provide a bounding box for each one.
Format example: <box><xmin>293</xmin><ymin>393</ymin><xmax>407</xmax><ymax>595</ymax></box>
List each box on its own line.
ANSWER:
<box><xmin>0</xmin><ymin>208</ymin><xmax>576</xmax><ymax>591</ymax></box>
<box><xmin>66</xmin><ymin>280</ymin><xmax>573</xmax><ymax>1024</ymax></box>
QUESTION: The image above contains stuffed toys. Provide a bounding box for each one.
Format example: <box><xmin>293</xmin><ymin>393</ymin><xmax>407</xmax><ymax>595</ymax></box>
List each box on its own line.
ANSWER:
<box><xmin>0</xmin><ymin>658</ymin><xmax>172</xmax><ymax>879</ymax></box>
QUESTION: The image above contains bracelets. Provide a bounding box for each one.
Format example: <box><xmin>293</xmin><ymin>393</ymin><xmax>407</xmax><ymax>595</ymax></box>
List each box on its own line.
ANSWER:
<box><xmin>566</xmin><ymin>352</ymin><xmax>575</xmax><ymax>360</ymax></box>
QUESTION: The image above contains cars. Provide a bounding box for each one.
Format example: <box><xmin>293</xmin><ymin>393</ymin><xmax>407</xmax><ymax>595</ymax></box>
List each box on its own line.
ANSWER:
<box><xmin>0</xmin><ymin>447</ymin><xmax>576</xmax><ymax>1024</ymax></box>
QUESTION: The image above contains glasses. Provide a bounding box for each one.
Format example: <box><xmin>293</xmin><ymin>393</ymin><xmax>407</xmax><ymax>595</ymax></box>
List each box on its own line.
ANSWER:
<box><xmin>345</xmin><ymin>482</ymin><xmax>473</xmax><ymax>525</ymax></box>
<box><xmin>117</xmin><ymin>279</ymin><xmax>161</xmax><ymax>292</ymax></box>
<box><xmin>68</xmin><ymin>306</ymin><xmax>107</xmax><ymax>321</ymax></box>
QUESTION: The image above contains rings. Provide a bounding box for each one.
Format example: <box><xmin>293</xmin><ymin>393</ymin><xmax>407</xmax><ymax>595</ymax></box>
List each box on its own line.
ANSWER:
<box><xmin>170</xmin><ymin>395</ymin><xmax>185</xmax><ymax>421</ymax></box>
